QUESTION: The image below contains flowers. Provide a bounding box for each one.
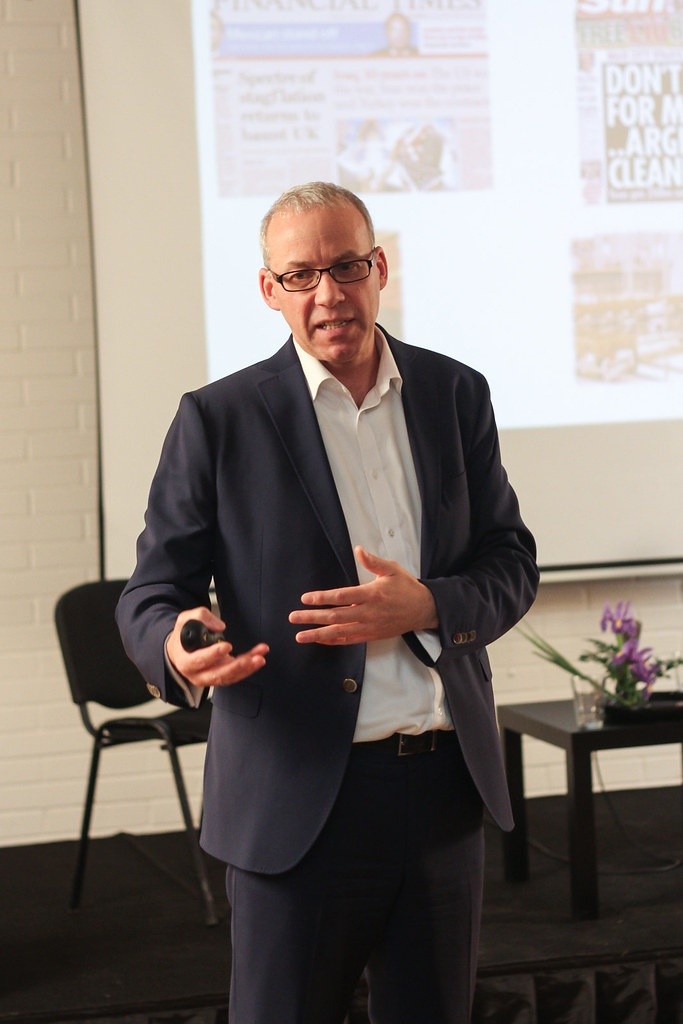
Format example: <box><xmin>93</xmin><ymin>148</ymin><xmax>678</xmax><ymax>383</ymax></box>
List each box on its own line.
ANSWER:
<box><xmin>514</xmin><ymin>598</ymin><xmax>683</xmax><ymax>707</ymax></box>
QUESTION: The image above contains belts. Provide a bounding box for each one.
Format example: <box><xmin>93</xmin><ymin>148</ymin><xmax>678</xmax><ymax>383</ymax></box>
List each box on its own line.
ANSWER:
<box><xmin>363</xmin><ymin>729</ymin><xmax>458</xmax><ymax>756</ymax></box>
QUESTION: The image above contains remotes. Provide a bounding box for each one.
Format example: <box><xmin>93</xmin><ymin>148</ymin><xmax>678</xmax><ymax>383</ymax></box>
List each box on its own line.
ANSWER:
<box><xmin>180</xmin><ymin>620</ymin><xmax>226</xmax><ymax>653</ymax></box>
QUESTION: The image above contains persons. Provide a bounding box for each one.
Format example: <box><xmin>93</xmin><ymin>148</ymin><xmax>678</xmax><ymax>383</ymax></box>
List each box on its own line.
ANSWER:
<box><xmin>113</xmin><ymin>181</ymin><xmax>540</xmax><ymax>1024</ymax></box>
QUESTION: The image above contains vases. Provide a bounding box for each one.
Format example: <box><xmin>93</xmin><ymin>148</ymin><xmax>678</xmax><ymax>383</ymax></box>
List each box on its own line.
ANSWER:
<box><xmin>603</xmin><ymin>691</ymin><xmax>683</xmax><ymax>725</ymax></box>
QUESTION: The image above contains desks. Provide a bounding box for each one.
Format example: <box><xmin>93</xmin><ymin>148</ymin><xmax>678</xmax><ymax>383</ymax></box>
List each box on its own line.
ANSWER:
<box><xmin>496</xmin><ymin>699</ymin><xmax>683</xmax><ymax>924</ymax></box>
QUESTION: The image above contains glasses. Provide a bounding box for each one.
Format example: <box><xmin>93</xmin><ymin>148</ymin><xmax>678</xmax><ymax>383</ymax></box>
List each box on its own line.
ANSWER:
<box><xmin>271</xmin><ymin>251</ymin><xmax>378</xmax><ymax>292</ymax></box>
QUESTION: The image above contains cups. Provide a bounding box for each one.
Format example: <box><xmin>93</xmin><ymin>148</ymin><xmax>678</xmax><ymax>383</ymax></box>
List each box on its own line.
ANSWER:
<box><xmin>569</xmin><ymin>674</ymin><xmax>607</xmax><ymax>731</ymax></box>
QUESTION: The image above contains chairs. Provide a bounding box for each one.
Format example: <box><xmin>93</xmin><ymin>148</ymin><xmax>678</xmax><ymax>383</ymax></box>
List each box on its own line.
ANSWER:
<box><xmin>54</xmin><ymin>578</ymin><xmax>222</xmax><ymax>929</ymax></box>
